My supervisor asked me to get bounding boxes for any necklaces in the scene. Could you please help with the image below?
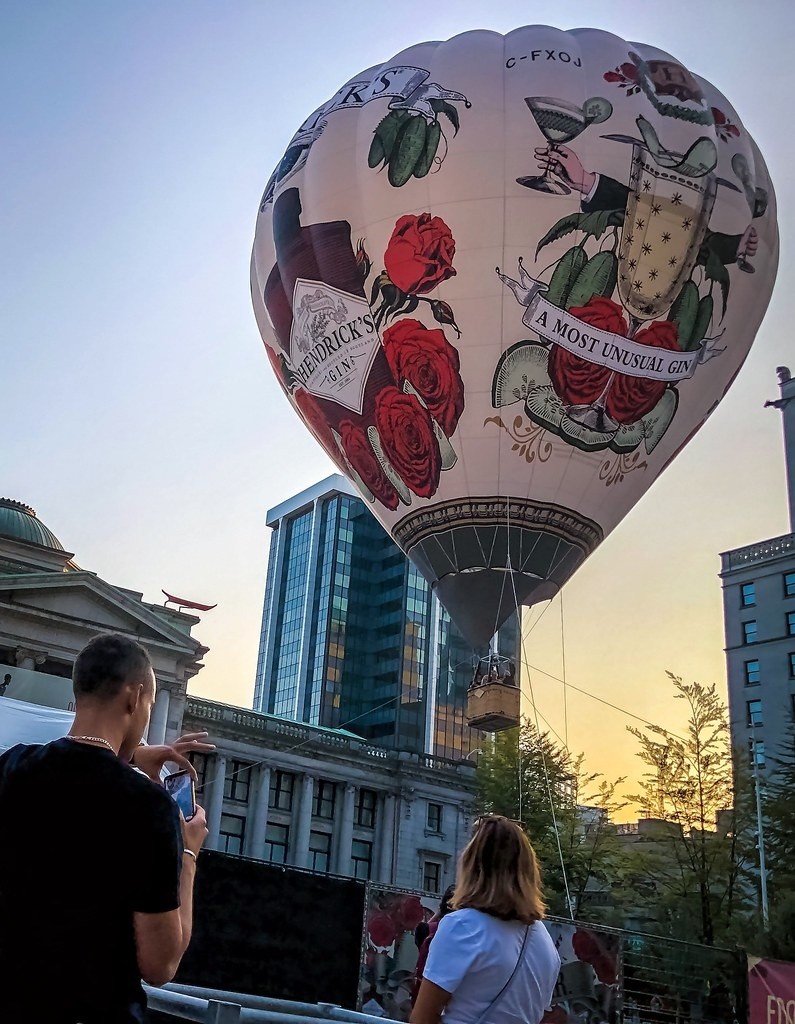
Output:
[66,735,114,751]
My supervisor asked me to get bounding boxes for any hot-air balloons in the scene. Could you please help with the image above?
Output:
[250,23,781,735]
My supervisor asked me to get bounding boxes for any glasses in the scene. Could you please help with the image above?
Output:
[477,815,526,837]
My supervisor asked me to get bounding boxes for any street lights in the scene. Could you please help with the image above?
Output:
[748,713,770,933]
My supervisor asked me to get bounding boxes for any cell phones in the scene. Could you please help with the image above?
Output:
[164,769,197,824]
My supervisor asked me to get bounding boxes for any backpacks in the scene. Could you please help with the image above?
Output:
[412,922,437,1011]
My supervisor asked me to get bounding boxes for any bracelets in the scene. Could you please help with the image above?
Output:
[183,848,197,862]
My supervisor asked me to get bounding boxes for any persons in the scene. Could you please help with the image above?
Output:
[469,659,516,687]
[0,634,215,1024]
[408,815,561,1024]
[412,884,457,1005]
